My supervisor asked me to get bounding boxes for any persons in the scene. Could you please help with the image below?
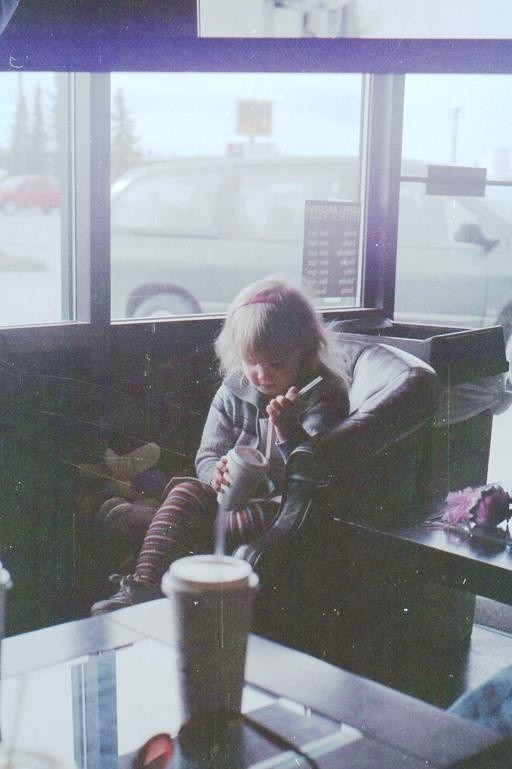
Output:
[87,274,348,616]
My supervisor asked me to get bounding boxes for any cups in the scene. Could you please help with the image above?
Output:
[159,554,258,750]
[213,447,268,512]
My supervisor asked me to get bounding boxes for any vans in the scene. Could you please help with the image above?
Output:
[105,157,512,383]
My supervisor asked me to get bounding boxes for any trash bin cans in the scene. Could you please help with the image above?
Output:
[327,316,509,492]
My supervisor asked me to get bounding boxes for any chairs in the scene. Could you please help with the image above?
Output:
[99,332,442,602]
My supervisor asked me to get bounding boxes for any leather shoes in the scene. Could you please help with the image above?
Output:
[90,574,159,614]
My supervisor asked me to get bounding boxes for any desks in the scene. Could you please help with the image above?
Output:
[0,598,504,769]
[334,487,512,680]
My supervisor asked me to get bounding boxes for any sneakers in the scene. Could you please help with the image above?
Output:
[103,503,160,533]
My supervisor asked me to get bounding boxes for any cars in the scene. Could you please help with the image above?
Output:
[1,173,57,212]
